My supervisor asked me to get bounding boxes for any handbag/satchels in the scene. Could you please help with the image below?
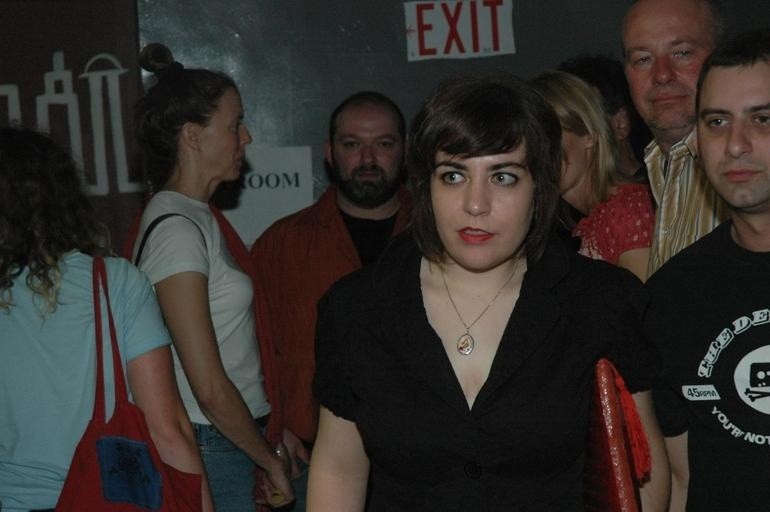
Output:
[56,401,203,512]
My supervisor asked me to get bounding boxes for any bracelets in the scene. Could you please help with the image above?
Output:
[271,448,288,454]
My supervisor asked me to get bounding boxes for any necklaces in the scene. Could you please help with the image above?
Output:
[435,254,521,357]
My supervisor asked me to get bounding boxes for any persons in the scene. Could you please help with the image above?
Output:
[305,76,670,510]
[559,53,652,199]
[524,66,654,287]
[250,90,415,510]
[131,41,296,511]
[644,29,770,510]
[621,0,729,283]
[0,125,216,512]
